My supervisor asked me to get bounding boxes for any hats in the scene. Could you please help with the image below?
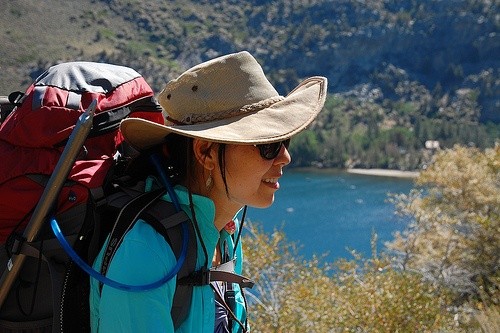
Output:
[118,48,327,156]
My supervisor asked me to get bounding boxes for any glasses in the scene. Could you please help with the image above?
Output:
[252,136,291,160]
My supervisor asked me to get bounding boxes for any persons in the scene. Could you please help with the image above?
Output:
[82,51,328,333]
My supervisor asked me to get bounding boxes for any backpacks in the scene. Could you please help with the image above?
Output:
[0,61,199,333]
